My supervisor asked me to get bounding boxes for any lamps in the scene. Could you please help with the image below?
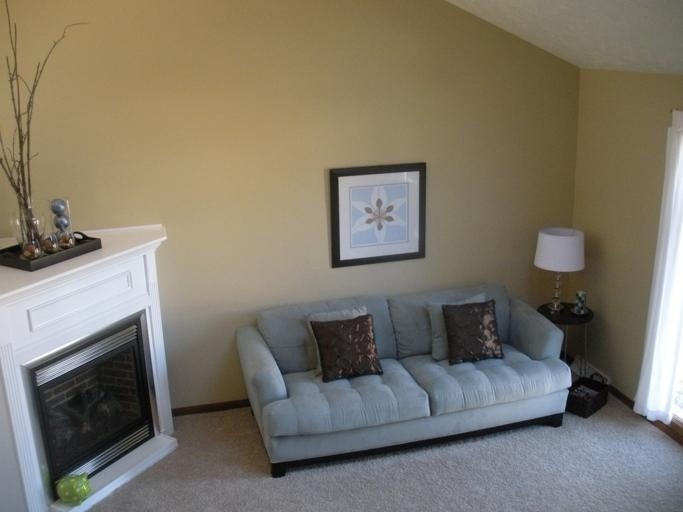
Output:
[532,226,586,317]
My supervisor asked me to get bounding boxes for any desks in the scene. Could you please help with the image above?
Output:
[538,299,592,368]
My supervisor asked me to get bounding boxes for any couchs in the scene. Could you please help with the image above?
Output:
[234,282,576,477]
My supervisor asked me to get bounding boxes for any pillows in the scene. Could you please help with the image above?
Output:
[441,298,503,367]
[310,311,384,381]
[422,293,486,361]
[304,304,366,379]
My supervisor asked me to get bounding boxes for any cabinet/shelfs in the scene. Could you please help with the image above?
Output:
[0,225,180,512]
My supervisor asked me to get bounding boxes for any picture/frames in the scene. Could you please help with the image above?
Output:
[328,162,428,269]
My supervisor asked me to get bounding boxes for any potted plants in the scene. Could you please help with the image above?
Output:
[10,192,46,256]
